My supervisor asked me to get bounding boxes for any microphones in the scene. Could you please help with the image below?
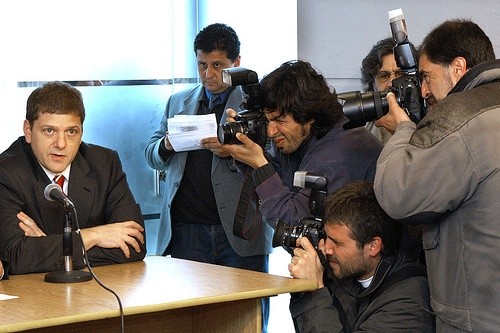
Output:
[44,183,75,209]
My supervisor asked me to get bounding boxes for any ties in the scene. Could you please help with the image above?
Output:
[209,95,220,109]
[53,175,65,193]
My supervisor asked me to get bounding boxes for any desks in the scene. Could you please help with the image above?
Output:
[0,255,319,333]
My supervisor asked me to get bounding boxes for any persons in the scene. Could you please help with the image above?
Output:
[290,19,500,333]
[222,60,385,259]
[0,22,275,333]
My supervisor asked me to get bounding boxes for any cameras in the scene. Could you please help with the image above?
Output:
[336,8,428,130]
[272,171,329,253]
[217,67,268,149]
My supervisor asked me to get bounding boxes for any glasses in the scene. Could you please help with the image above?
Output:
[376,69,402,82]
